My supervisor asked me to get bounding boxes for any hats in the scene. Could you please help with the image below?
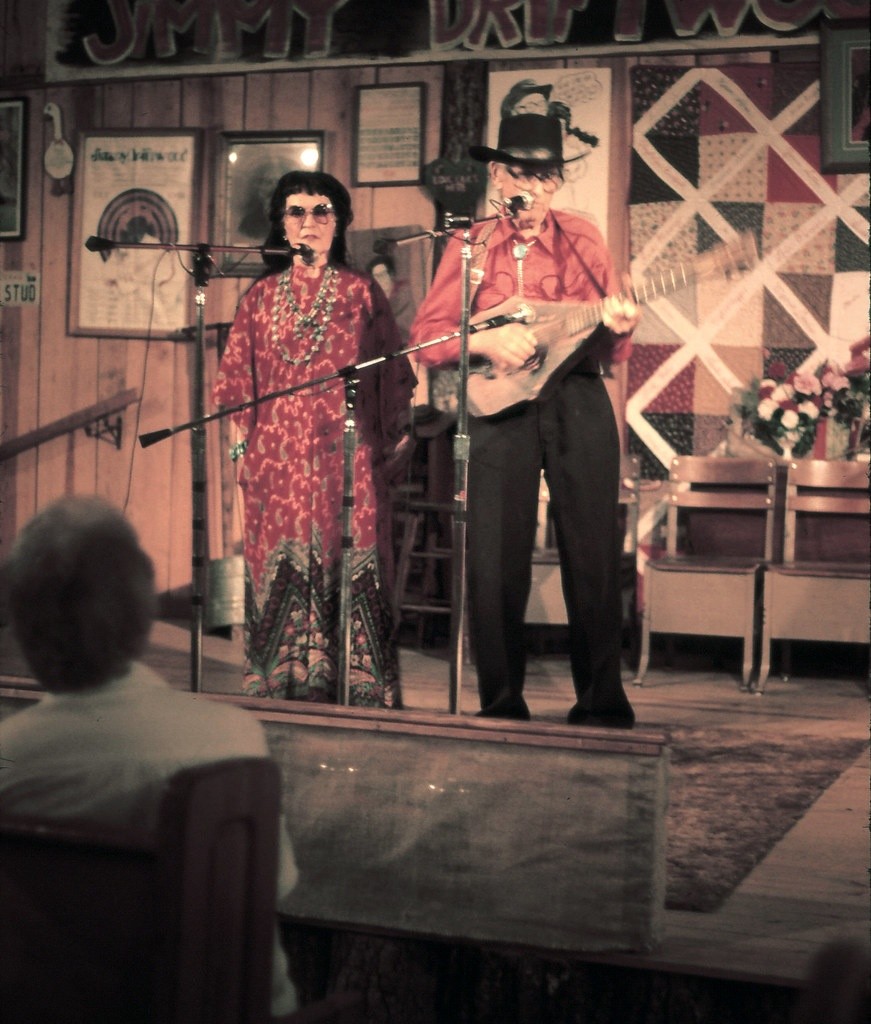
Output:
[470,113,591,168]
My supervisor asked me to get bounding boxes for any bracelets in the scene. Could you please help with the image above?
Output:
[229,440,248,463]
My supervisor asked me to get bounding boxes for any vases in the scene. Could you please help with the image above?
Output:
[773,429,800,458]
[848,422,862,457]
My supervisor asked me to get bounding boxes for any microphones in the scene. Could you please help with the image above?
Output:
[503,191,535,211]
[473,306,536,333]
[300,243,319,266]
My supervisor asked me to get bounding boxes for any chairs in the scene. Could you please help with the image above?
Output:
[632,455,777,689]
[756,458,871,693]
[510,461,643,626]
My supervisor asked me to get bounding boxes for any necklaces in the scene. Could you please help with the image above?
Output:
[511,236,537,296]
[271,265,338,364]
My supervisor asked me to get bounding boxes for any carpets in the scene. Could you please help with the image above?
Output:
[630,719,871,917]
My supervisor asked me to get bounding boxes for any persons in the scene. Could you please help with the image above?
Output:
[212,169,420,709]
[0,495,294,1024]
[411,116,638,730]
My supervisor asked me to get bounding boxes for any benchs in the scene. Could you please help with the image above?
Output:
[0,755,289,1024]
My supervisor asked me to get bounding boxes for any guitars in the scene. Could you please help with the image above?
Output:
[429,233,755,418]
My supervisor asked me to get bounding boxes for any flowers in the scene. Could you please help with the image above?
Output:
[795,339,870,415]
[750,377,821,439]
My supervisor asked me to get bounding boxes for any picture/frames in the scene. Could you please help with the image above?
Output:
[0,99,31,240]
[819,19,870,176]
[211,129,329,279]
[65,123,210,343]
[350,81,429,191]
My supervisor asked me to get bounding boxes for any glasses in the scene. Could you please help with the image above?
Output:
[505,165,564,194]
[284,203,335,223]
